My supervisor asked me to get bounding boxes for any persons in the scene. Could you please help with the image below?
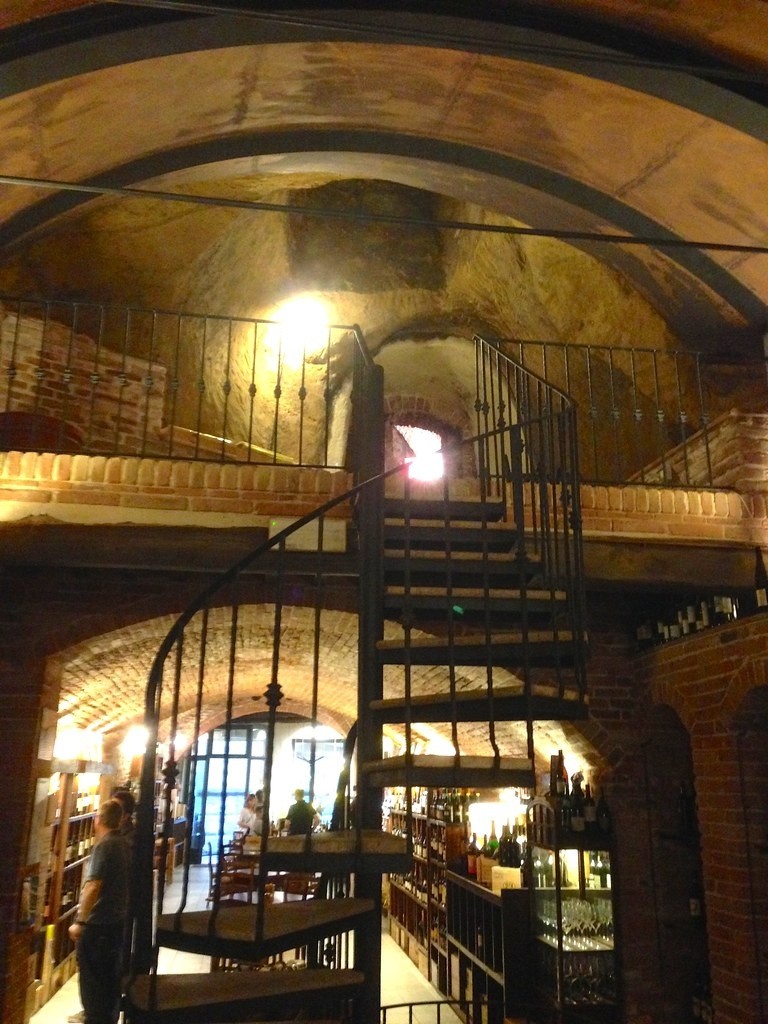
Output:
[237,789,264,839]
[67,792,141,1024]
[285,789,320,835]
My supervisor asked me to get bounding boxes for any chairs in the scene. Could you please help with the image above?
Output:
[208,830,320,905]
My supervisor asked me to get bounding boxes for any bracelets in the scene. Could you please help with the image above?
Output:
[73,920,86,925]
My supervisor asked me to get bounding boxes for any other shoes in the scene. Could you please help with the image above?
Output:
[68,1011,86,1023]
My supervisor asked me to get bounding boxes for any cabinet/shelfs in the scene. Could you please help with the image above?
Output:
[387,774,628,1024]
[33,758,116,1015]
